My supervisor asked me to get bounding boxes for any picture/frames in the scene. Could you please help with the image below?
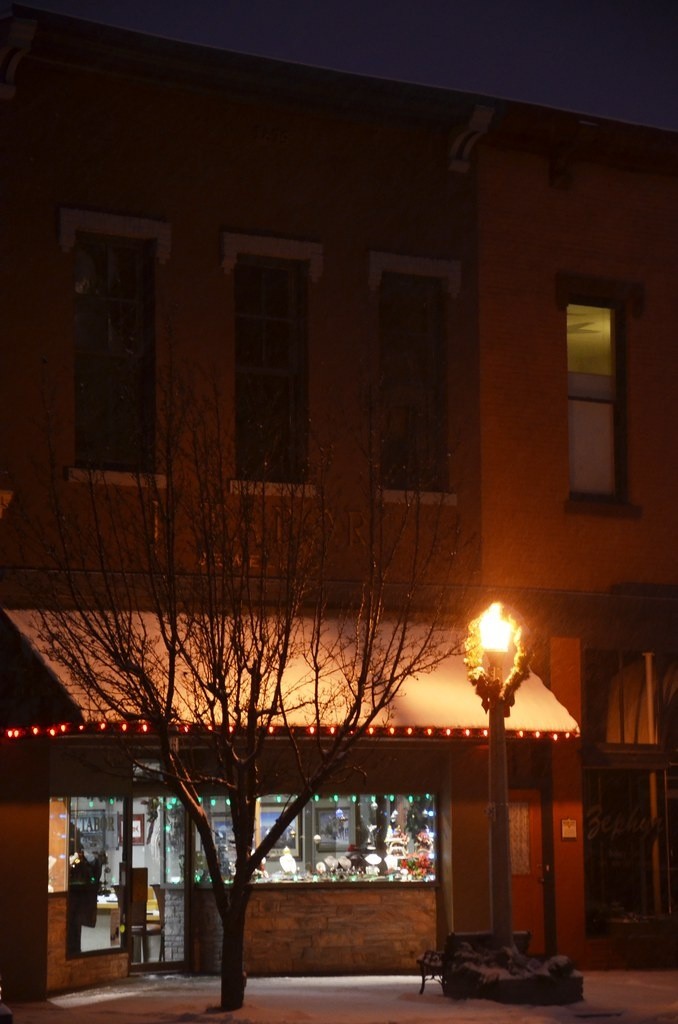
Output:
[255,802,302,861]
[117,812,145,846]
[207,812,237,862]
[314,807,361,852]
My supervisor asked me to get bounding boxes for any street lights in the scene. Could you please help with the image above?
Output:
[473,597,514,954]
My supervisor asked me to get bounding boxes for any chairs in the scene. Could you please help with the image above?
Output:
[111,885,162,962]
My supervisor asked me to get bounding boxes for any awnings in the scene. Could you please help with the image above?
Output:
[582,753,671,769]
[0,608,582,740]
[583,583,678,654]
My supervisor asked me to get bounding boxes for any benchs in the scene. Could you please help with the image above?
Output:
[417,930,531,997]
